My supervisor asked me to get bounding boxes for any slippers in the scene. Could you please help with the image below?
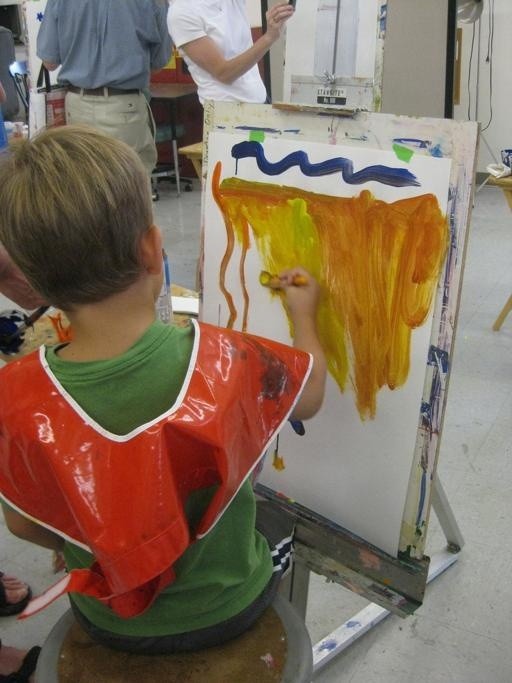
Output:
[0,640,41,683]
[0,571,32,617]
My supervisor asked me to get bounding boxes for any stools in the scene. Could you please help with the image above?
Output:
[32,591,315,683]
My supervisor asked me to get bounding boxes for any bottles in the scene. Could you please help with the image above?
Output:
[157,249,172,324]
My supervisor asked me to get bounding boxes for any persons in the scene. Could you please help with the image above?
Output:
[36,0,173,177]
[0,82,54,681]
[1,127,326,657]
[0,26,20,122]
[167,0,303,139]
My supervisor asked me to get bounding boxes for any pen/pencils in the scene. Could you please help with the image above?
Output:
[270,276,308,288]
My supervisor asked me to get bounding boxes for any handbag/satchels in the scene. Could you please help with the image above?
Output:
[28,63,67,139]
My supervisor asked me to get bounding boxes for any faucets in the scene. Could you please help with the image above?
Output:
[320,71,335,87]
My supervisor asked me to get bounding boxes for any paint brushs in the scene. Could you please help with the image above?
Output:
[3,306,49,344]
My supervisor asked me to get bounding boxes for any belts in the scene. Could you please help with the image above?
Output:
[67,84,139,96]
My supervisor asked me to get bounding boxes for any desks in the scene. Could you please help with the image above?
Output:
[149,81,197,196]
